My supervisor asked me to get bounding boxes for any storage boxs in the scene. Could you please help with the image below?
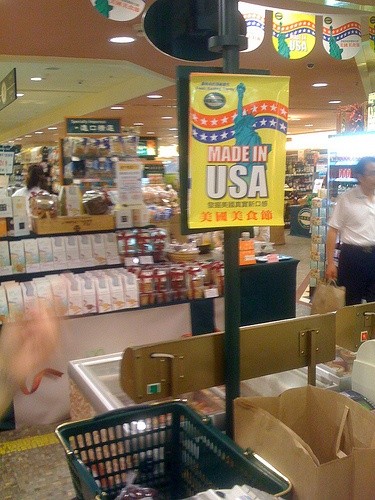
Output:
[31,214,116,234]
[233,384,375,500]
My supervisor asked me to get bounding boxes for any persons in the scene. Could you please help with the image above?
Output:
[0,294,64,417]
[11,165,50,230]
[326,157,375,306]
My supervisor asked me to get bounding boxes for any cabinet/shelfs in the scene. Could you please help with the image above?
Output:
[284,152,328,229]
[324,129,375,283]
[0,225,223,429]
